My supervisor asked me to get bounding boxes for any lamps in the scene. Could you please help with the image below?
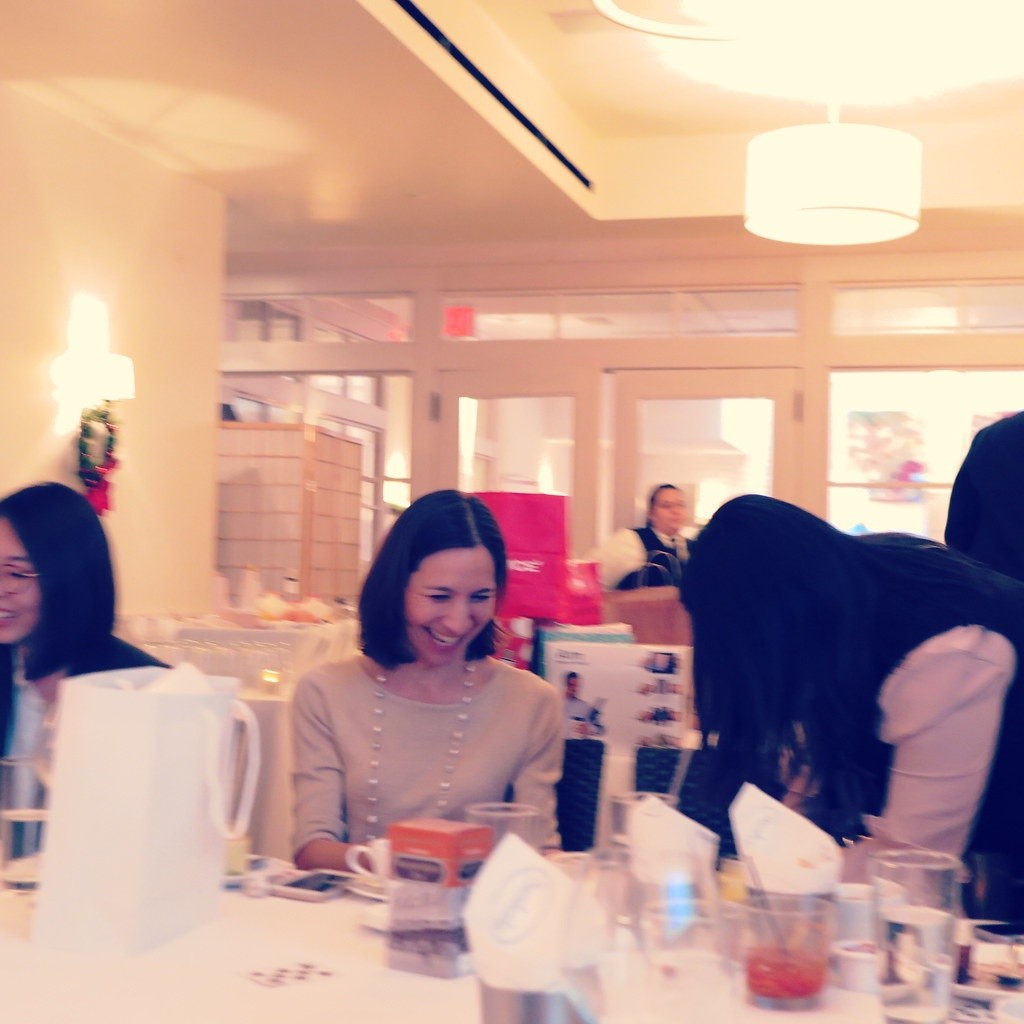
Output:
[743,107,924,245]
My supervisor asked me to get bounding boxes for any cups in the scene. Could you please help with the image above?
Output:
[0,755,46,891]
[464,801,543,855]
[603,792,679,916]
[994,995,1024,1024]
[836,882,876,937]
[876,905,954,984]
[346,837,391,878]
[744,896,837,1012]
[869,849,961,1024]
[647,896,744,1024]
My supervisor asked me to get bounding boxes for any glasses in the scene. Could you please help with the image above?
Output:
[0,564,45,595]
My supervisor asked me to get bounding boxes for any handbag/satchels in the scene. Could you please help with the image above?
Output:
[604,549,692,646]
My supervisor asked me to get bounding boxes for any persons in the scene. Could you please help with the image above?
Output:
[0,482,173,867]
[600,412,1024,936]
[287,491,565,875]
[561,671,606,741]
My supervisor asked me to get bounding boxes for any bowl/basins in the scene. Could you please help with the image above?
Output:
[830,939,956,989]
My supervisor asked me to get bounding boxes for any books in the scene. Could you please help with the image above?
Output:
[534,620,693,751]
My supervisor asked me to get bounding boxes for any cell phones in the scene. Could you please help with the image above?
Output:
[274,870,355,902]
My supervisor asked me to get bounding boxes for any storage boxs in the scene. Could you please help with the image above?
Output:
[387,819,496,981]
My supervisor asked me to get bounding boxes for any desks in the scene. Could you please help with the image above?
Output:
[0,865,1024,1024]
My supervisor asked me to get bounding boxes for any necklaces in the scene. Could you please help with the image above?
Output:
[365,656,476,845]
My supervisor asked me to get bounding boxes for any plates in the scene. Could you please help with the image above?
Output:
[339,882,391,901]
[219,855,292,887]
[255,620,295,630]
[947,918,1024,1000]
